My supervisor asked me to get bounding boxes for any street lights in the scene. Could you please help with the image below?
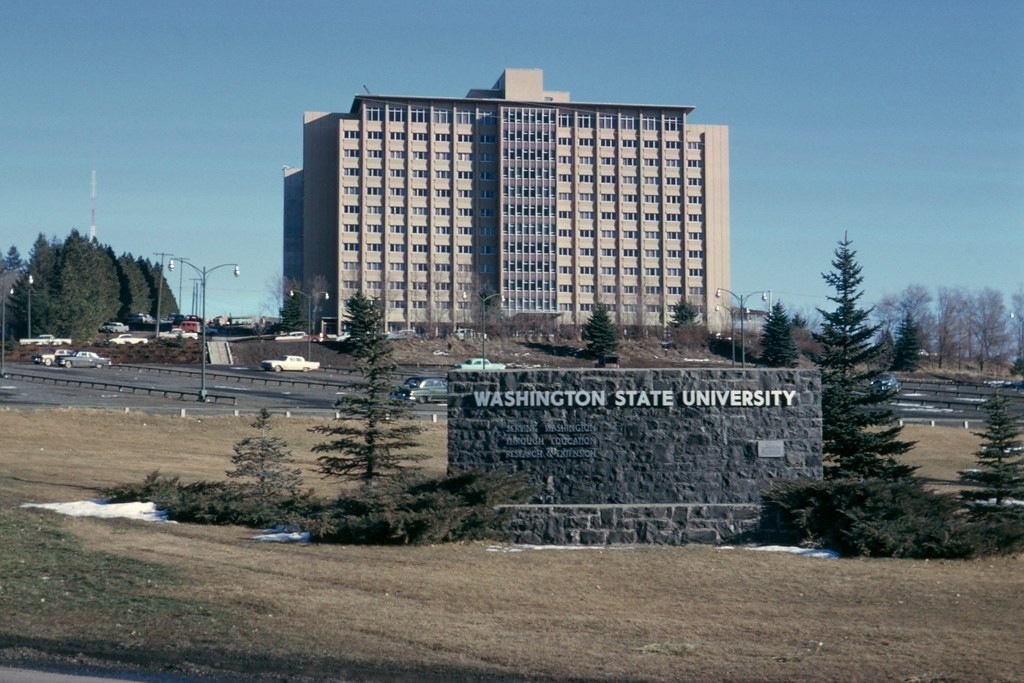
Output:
[289,290,330,361]
[167,257,240,402]
[460,290,505,370]
[713,287,767,367]
[9,273,33,338]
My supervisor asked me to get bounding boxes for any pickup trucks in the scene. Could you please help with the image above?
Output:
[108,333,149,344]
[159,327,198,341]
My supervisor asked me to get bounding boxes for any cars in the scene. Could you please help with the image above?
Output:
[261,355,321,371]
[869,373,903,397]
[200,324,219,335]
[335,332,352,342]
[31,349,112,368]
[99,322,130,333]
[452,328,487,341]
[389,376,447,404]
[134,312,198,324]
[39,334,55,340]
[275,330,309,341]
[453,358,506,372]
